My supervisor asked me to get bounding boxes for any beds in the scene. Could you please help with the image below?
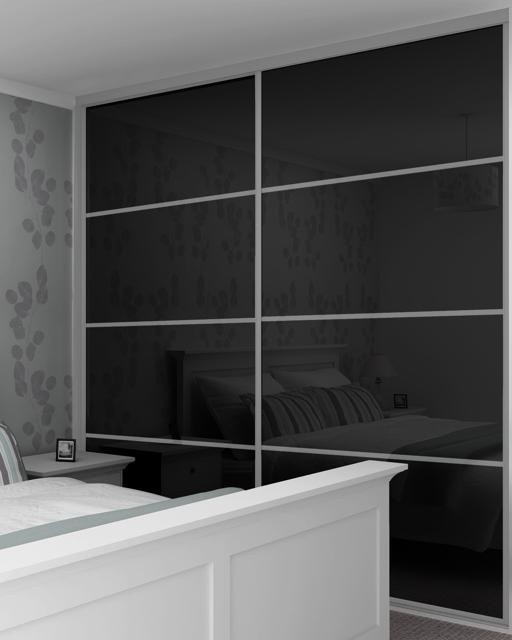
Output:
[0,433,409,639]
[167,343,500,492]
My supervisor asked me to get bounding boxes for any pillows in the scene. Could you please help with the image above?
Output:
[196,369,383,435]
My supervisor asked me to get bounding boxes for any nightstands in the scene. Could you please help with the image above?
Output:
[100,434,230,498]
[383,406,431,419]
[21,450,135,487]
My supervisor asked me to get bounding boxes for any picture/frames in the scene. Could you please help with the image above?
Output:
[394,393,408,410]
[55,437,76,461]
